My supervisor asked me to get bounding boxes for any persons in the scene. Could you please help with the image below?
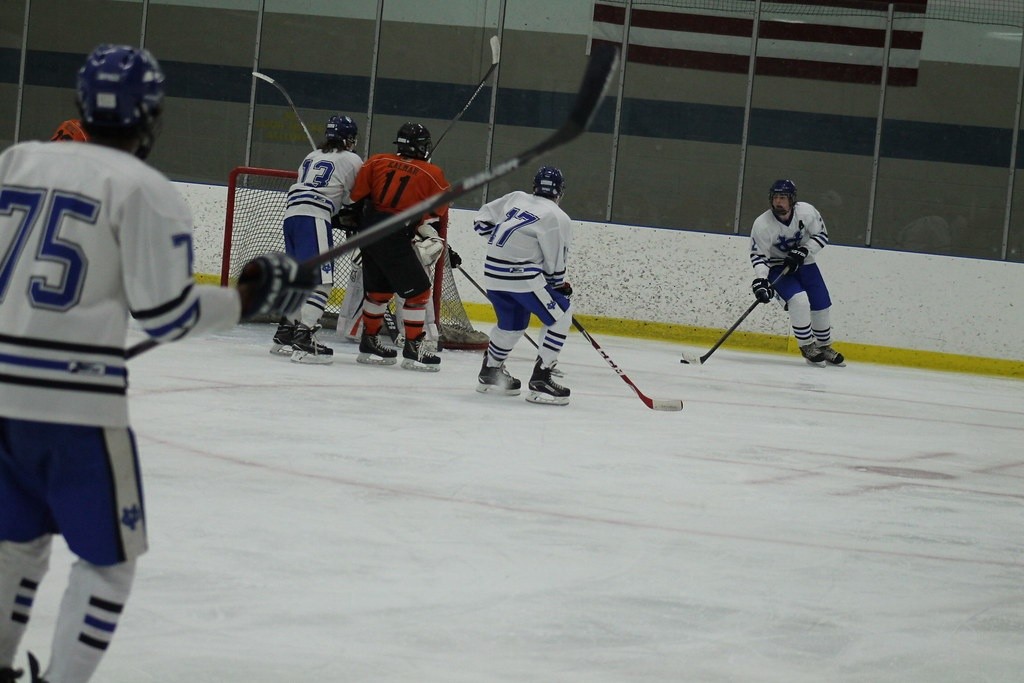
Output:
[270,115,363,364]
[351,122,450,372]
[0,43,309,683]
[51,119,88,143]
[473,166,574,405]
[751,180,847,368]
[337,215,461,352]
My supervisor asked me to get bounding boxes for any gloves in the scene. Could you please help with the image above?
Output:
[752,278,774,304]
[446,243,462,268]
[555,282,573,297]
[237,251,319,324]
[782,246,808,276]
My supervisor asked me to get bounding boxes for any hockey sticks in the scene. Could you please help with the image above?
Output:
[123,39,626,363]
[456,264,541,351]
[571,316,685,412]
[681,267,790,366]
[251,70,318,151]
[425,35,503,162]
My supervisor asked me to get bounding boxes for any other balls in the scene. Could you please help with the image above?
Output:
[680,360,689,364]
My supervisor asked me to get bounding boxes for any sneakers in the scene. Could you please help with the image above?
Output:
[401,331,441,372]
[799,342,827,367]
[820,345,847,367]
[291,322,334,364]
[355,325,397,365]
[476,351,522,395]
[270,316,299,355]
[526,359,571,405]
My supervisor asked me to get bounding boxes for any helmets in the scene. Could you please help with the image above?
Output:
[324,115,359,152]
[533,166,565,199]
[768,179,798,214]
[77,42,180,150]
[393,121,433,160]
